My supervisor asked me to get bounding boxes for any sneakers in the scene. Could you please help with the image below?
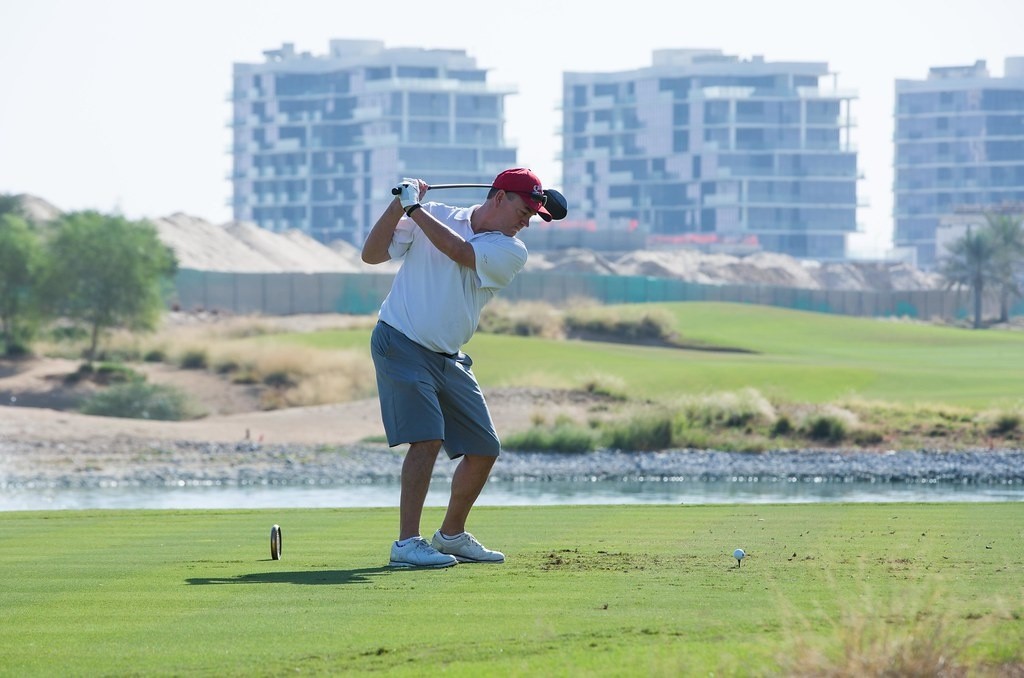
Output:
[388,537,458,569]
[431,528,505,563]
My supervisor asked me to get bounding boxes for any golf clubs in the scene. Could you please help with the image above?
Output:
[392,183,567,221]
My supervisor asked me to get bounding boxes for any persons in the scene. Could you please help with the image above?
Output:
[360,167,548,569]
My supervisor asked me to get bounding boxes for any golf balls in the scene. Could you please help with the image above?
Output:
[734,549,745,560]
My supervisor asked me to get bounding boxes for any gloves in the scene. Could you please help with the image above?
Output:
[395,177,428,212]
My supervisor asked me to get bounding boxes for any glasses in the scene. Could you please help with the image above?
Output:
[505,189,548,206]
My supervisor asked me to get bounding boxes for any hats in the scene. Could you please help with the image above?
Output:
[492,168,553,223]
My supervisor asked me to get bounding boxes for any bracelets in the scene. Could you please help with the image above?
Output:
[406,203,422,218]
